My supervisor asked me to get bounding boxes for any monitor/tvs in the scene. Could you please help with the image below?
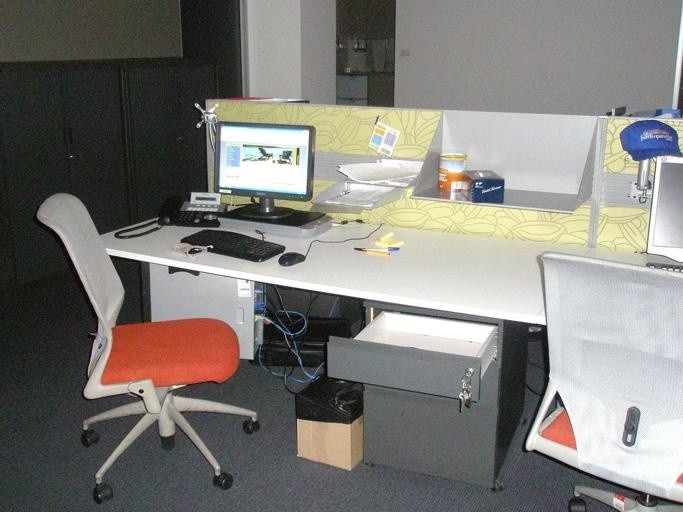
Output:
[648,156,683,262]
[215,120,326,227]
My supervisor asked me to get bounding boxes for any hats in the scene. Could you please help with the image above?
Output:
[619,119,683,160]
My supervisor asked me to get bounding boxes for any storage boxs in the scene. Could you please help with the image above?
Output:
[296,378,363,471]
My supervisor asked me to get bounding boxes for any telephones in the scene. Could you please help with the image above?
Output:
[115,192,228,239]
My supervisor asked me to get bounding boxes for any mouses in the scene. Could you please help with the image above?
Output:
[278,252,305,265]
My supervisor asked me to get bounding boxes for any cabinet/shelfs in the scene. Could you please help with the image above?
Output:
[0,59,131,286]
[337,73,393,107]
[124,59,217,225]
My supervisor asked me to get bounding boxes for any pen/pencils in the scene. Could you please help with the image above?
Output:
[362,247,400,251]
[354,248,390,256]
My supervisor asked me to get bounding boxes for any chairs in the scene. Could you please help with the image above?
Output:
[524,251,683,512]
[36,192,260,503]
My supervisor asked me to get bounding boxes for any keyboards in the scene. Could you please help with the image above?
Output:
[645,263,681,272]
[180,229,285,262]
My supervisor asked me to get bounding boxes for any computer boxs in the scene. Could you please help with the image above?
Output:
[140,260,267,361]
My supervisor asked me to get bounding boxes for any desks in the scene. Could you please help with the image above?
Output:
[99,97,683,488]
